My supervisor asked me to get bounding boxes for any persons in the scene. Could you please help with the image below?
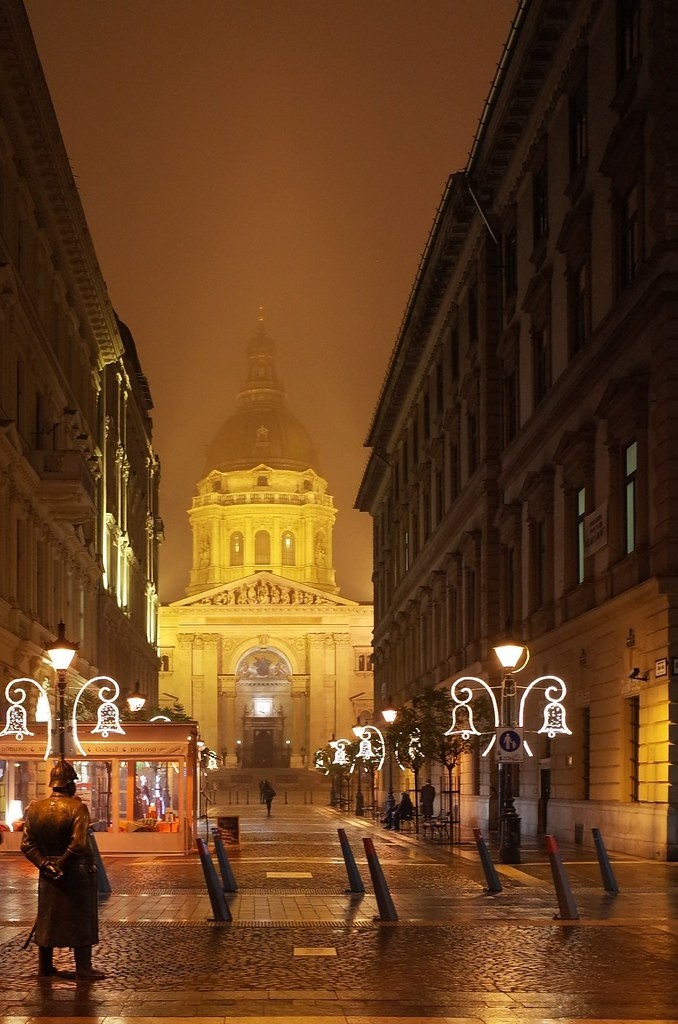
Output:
[258,780,265,802]
[392,792,413,830]
[380,803,401,829]
[264,780,268,784]
[264,782,274,815]
[420,779,436,821]
[19,759,106,981]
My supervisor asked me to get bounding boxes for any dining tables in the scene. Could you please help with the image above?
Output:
[0,810,180,833]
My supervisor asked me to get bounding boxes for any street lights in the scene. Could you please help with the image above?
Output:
[0,618,126,760]
[444,643,574,861]
[126,679,170,723]
[197,734,219,818]
[315,734,349,807]
[353,708,427,834]
[329,716,381,815]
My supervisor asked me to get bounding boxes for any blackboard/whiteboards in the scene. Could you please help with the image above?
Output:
[215,815,241,846]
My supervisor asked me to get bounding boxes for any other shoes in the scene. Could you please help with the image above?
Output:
[378,814,437,833]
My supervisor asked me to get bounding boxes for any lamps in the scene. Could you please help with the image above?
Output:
[285,534,291,547]
[234,536,240,553]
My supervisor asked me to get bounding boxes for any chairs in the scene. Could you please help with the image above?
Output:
[331,797,450,839]
